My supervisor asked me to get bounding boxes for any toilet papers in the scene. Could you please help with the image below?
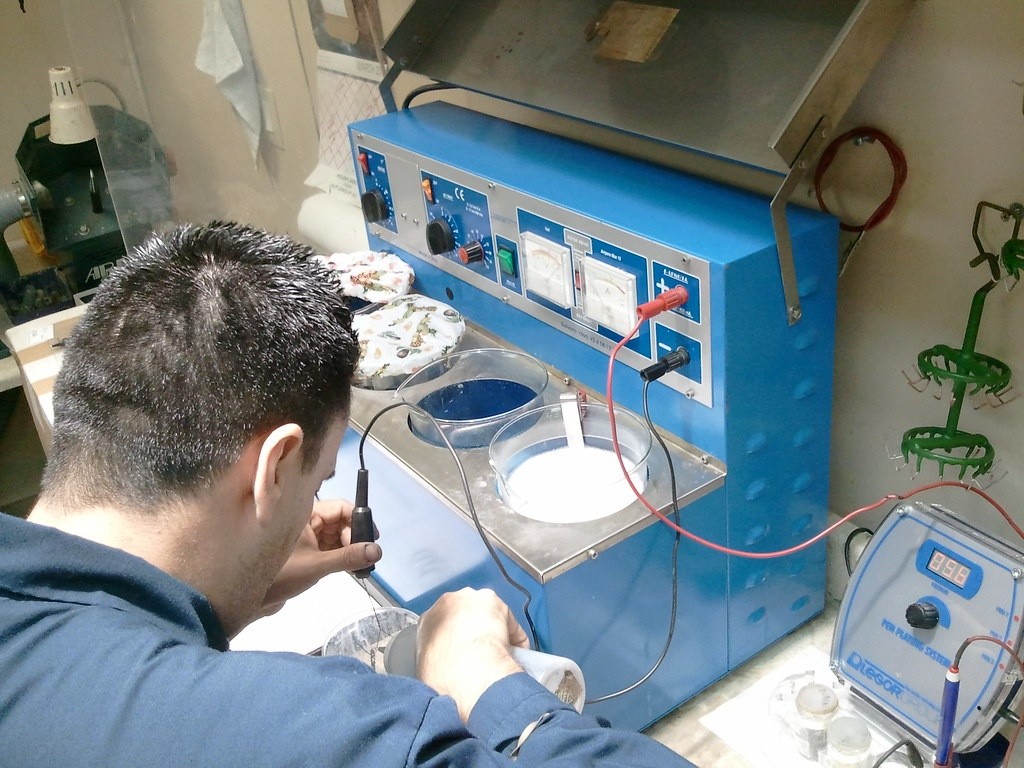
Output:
[295,191,370,255]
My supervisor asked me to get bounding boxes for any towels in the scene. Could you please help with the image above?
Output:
[192,0,265,175]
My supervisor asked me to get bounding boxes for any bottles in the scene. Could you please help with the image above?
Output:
[379,625,586,718]
[792,684,871,768]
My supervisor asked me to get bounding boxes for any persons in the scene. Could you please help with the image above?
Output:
[0,220,699,767]
